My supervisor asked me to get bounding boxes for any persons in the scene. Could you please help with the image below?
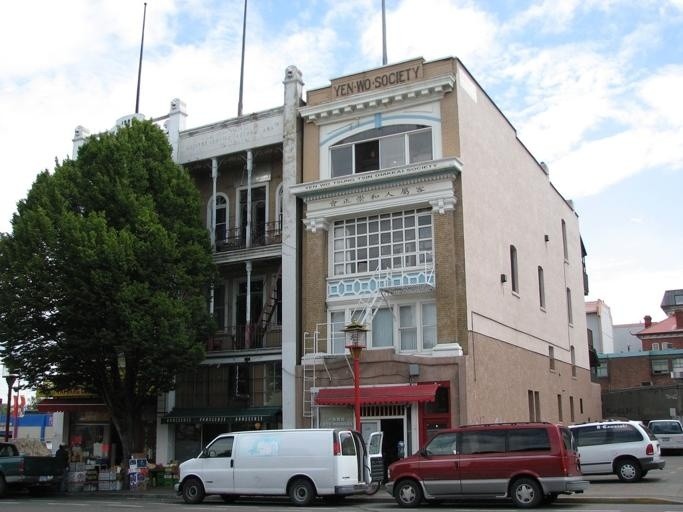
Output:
[56,442,71,492]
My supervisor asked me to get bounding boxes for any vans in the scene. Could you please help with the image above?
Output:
[385,422,590,508]
[567,419,666,481]
[174,429,384,507]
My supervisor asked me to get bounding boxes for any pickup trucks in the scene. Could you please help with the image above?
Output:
[0,443,65,496]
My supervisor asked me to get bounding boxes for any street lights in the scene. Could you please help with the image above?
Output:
[342,320,368,437]
[2,375,18,441]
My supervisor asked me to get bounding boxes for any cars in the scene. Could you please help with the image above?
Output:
[647,418,683,449]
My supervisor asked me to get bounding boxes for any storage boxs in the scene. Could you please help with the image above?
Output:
[64,458,149,491]
